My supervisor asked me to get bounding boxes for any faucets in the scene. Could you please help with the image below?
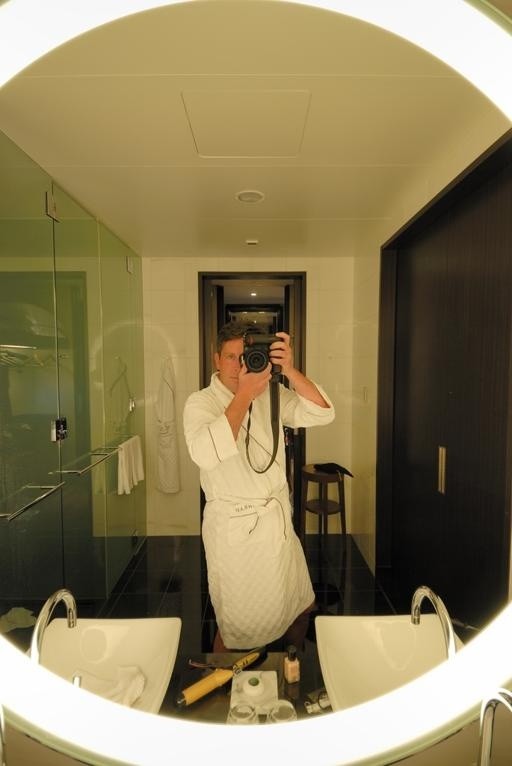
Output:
[413,586,456,659]
[475,689,512,765]
[28,587,79,662]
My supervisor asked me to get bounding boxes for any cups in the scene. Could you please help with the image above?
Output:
[226,702,259,724]
[266,699,298,724]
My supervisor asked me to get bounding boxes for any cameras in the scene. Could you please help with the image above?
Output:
[239,332,284,373]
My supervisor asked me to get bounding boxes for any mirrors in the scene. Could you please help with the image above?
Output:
[0,0,512,766]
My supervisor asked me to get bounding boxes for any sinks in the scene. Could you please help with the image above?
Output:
[32,618,180,714]
[313,612,464,712]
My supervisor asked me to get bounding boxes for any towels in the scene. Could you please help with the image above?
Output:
[66,665,145,708]
[117,435,145,495]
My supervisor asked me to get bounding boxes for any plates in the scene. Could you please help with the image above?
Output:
[230,670,279,715]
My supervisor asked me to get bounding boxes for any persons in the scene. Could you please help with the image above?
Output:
[184,319,336,654]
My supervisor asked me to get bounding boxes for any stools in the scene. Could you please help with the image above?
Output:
[300,464,347,549]
